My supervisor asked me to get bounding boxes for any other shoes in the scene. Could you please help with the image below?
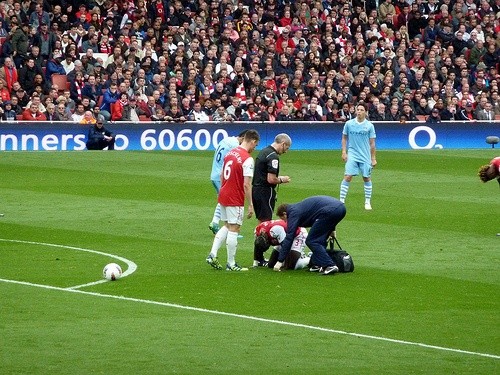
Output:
[364,203,372,210]
[258,259,269,266]
[308,252,313,257]
[301,252,306,258]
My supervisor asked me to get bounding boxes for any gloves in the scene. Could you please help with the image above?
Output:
[273,261,282,272]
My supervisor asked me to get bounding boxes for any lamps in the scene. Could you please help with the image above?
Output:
[486,135,499,149]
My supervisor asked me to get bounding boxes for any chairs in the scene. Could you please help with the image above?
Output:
[51,73,66,91]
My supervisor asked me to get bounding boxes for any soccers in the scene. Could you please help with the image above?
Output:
[103,263,122,280]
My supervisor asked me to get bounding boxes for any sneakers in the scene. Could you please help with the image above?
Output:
[322,266,339,275]
[226,263,248,272]
[209,222,220,234]
[309,265,322,272]
[206,253,223,270]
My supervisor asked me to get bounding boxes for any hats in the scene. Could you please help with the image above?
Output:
[270,225,286,243]
[466,102,472,107]
[185,90,193,94]
[97,118,103,124]
[254,235,270,252]
[433,108,439,113]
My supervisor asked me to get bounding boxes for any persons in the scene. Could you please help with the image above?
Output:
[273,195,346,276]
[0,0,500,122]
[252,134,292,267]
[208,129,249,239]
[86,119,115,151]
[478,156,500,236]
[254,219,313,270]
[339,103,378,210]
[207,129,261,272]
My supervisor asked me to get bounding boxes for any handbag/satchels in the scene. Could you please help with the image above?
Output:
[325,236,354,273]
